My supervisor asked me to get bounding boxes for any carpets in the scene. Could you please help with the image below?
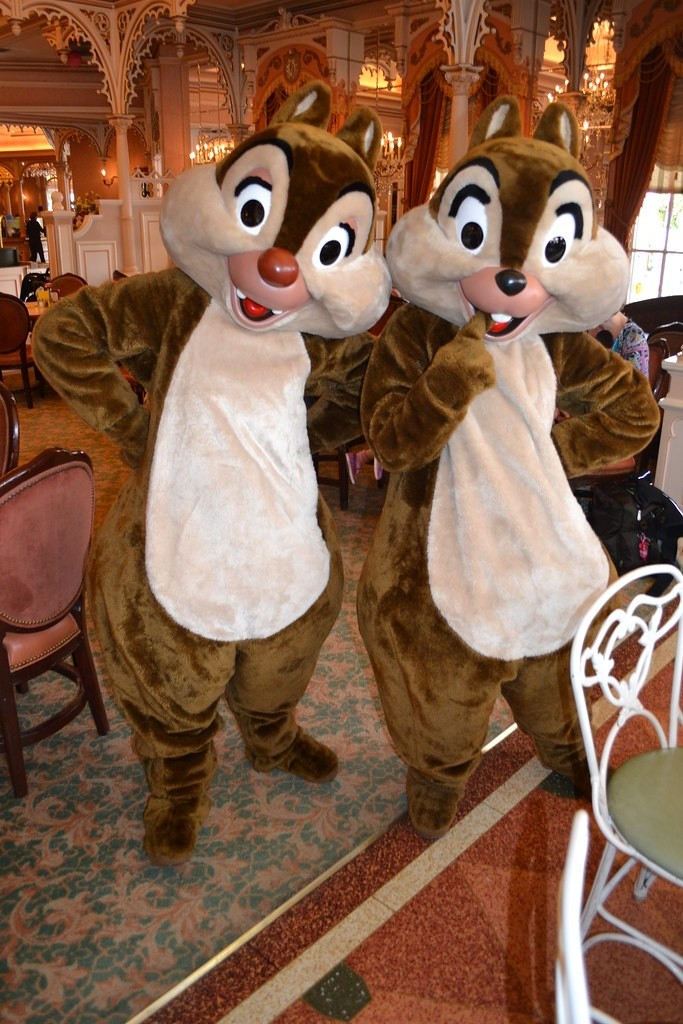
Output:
[124,592,682,1024]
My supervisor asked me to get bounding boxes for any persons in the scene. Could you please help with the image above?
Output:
[345,448,384,484]
[550,305,648,567]
[26,212,45,262]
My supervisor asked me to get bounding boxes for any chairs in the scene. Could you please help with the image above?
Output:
[551,564,683,1024]
[0,267,134,480]
[1,443,112,798]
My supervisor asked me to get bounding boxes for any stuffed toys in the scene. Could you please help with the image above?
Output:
[358,96,661,837]
[30,79,392,863]
